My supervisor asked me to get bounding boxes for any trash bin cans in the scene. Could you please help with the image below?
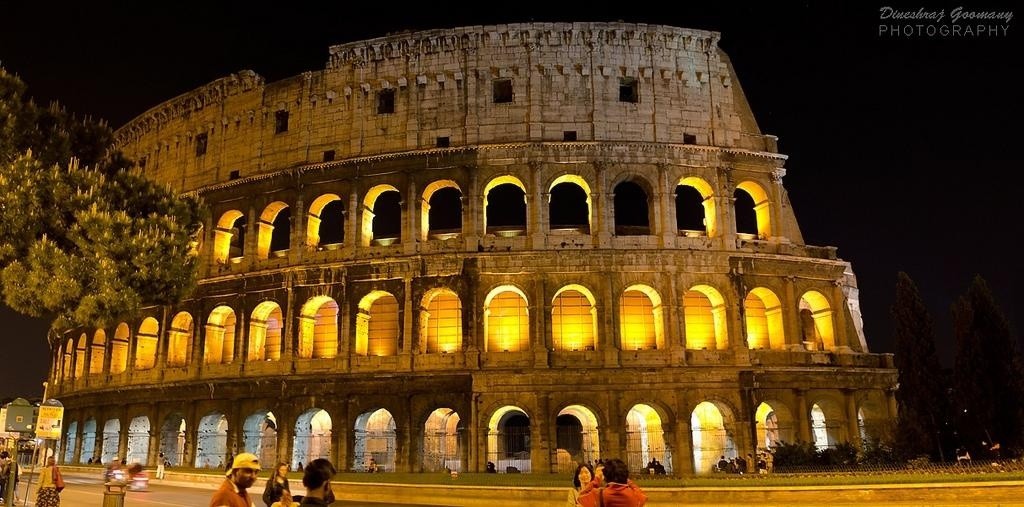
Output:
[103,482,126,507]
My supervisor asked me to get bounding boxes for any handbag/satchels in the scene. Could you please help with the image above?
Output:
[55,472,65,491]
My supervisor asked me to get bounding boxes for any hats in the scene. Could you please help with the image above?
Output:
[226,453,261,476]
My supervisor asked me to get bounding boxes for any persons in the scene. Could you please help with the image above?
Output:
[0,450,141,506]
[715,452,767,474]
[163,457,171,467]
[487,461,494,472]
[644,456,666,475]
[155,452,165,479]
[587,458,608,470]
[576,458,648,506]
[209,452,336,506]
[368,458,376,472]
[565,462,595,506]
